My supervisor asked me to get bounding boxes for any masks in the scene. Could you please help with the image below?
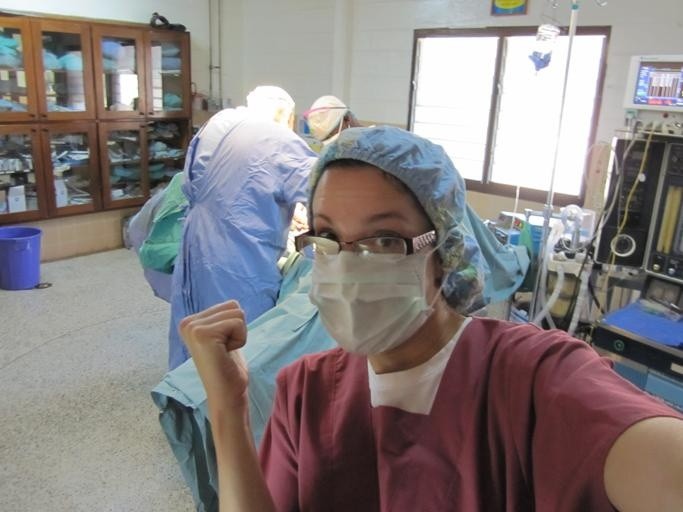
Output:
[307,248,435,357]
[321,133,340,152]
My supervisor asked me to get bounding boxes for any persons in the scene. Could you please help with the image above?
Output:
[298,95,378,144]
[175,119,682,512]
[168,84,327,367]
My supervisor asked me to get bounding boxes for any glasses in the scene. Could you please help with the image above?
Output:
[293,229,437,268]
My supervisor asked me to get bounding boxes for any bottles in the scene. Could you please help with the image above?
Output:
[526,23,560,71]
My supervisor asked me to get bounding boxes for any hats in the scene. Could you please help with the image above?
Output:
[307,125,491,317]
[247,86,295,131]
[306,95,350,143]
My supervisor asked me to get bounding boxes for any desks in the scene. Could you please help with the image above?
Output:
[591,298,683,415]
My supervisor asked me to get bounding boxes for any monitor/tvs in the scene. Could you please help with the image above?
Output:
[624,55,683,112]
